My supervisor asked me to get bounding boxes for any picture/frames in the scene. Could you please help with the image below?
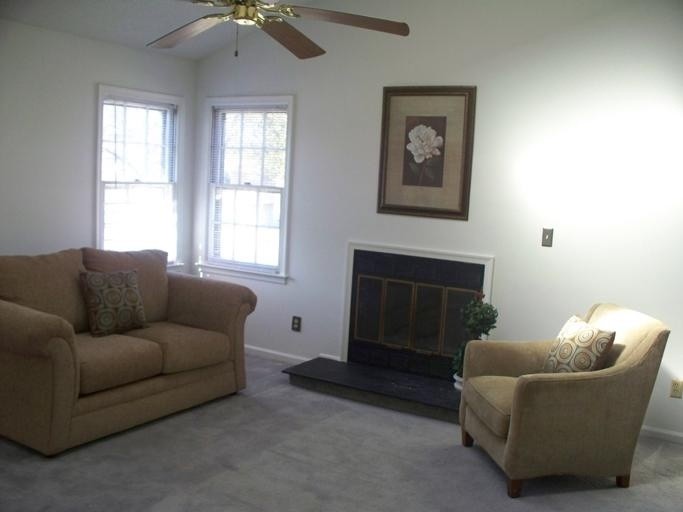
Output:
[376,84,477,221]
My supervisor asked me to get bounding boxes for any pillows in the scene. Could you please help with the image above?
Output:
[542,312,618,373]
[77,267,151,339]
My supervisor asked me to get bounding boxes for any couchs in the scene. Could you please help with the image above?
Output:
[1,249,258,458]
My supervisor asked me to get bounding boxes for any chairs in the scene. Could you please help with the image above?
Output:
[458,302,671,499]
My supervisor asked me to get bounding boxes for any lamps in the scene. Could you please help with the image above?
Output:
[231,3,268,29]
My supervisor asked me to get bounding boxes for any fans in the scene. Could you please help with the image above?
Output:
[144,2,411,59]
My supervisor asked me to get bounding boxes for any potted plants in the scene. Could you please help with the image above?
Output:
[450,300,498,392]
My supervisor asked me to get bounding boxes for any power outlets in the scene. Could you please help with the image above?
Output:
[670,378,683,398]
